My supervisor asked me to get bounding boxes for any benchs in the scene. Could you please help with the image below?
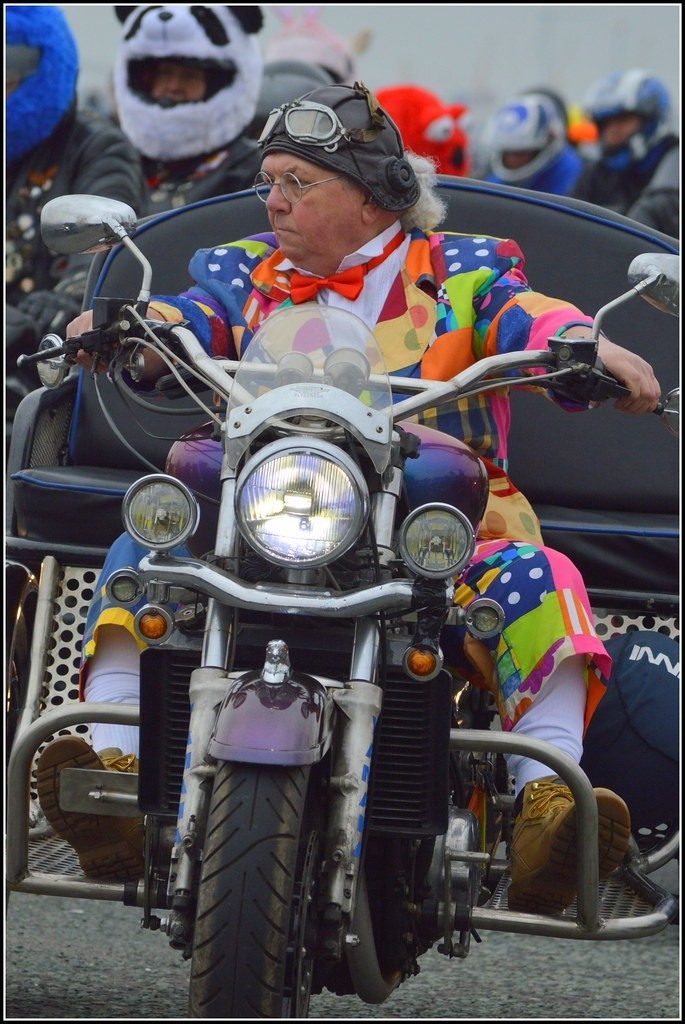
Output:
[11,171,680,598]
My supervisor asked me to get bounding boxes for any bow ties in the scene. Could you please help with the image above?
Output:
[288,229,405,305]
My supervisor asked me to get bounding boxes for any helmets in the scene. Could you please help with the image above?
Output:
[7,5,79,167]
[488,85,569,186]
[583,67,670,178]
[110,6,263,162]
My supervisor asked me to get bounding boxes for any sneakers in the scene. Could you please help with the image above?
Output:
[37,736,148,884]
[507,771,631,915]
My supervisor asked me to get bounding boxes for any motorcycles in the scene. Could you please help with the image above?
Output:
[6,174,681,1020]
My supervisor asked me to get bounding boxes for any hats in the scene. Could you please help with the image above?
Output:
[257,81,421,211]
[376,85,470,181]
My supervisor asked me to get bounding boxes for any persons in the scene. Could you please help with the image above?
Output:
[8,5,678,351]
[36,82,661,916]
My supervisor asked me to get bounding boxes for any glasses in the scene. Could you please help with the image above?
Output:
[254,100,346,147]
[251,171,345,204]
